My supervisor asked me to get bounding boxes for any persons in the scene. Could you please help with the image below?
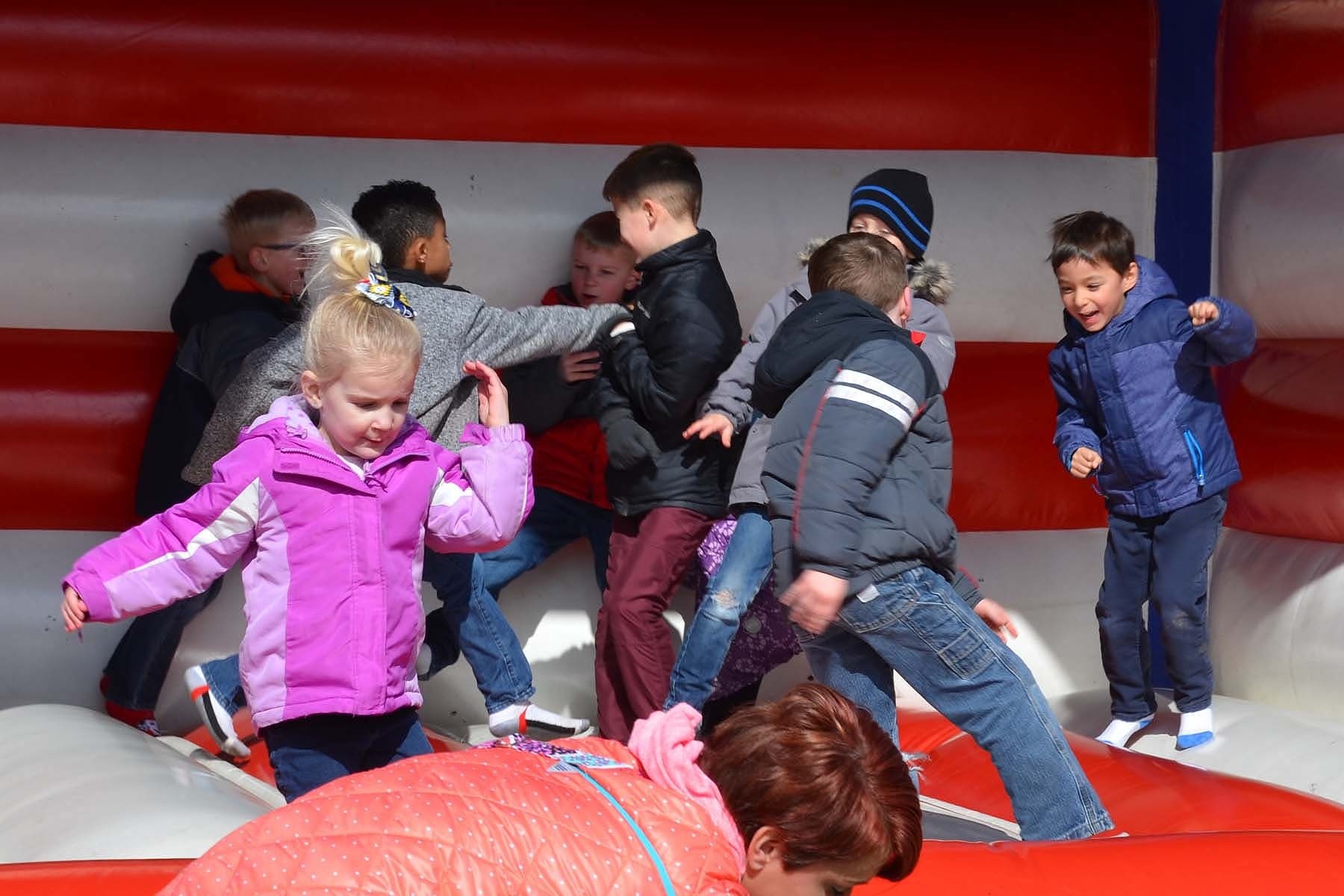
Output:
[753,231,1110,839]
[412,212,641,679]
[99,187,315,743]
[62,238,534,802]
[1048,210,1256,751]
[157,683,923,896]
[181,178,629,760]
[582,142,738,746]
[664,169,957,754]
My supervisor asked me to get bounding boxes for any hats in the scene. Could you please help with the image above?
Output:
[845,165,934,262]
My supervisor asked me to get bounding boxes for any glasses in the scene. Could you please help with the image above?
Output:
[253,244,313,261]
